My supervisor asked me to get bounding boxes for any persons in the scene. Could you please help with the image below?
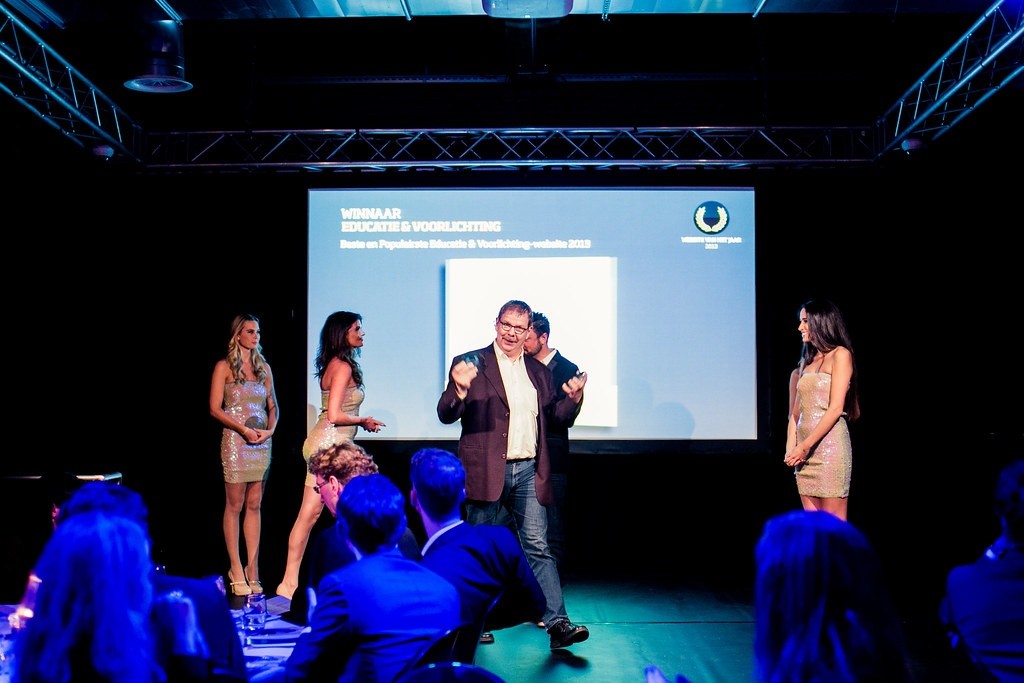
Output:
[276,312,386,600]
[942,464,1024,683]
[645,510,958,683]
[437,300,589,648]
[9,482,249,683]
[411,449,547,634]
[286,473,460,683]
[307,440,417,572]
[784,299,860,522]
[211,317,279,595]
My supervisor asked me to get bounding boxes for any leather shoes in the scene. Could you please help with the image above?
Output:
[479,631,493,641]
[549,621,589,648]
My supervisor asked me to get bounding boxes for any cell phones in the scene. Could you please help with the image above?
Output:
[576,372,585,378]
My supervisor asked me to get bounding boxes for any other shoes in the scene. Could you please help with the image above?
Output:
[537,620,545,626]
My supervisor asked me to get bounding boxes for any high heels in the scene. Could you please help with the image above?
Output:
[243,565,262,593]
[228,567,251,596]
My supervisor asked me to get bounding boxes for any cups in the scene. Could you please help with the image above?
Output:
[244,595,266,630]
[231,611,244,648]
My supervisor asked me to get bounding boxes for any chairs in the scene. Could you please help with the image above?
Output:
[396,593,507,683]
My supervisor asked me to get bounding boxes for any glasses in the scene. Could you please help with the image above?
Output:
[314,480,329,494]
[498,318,529,335]
